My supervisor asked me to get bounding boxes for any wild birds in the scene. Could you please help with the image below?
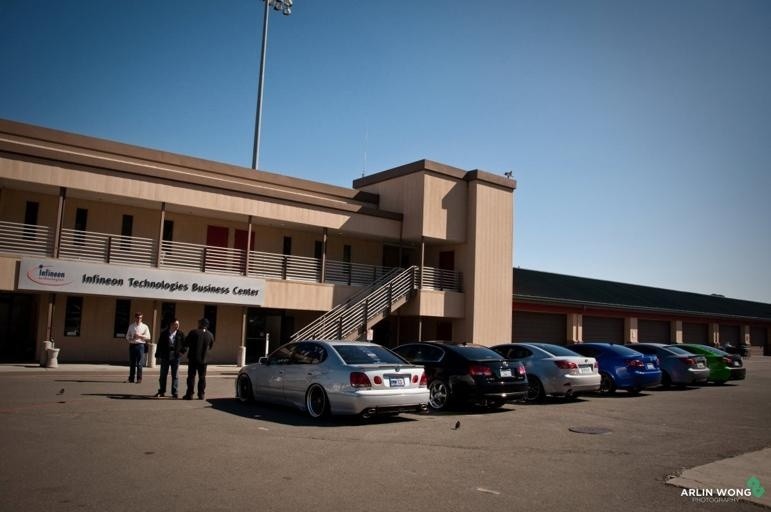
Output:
[455,421,460,431]
[60,388,64,394]
[505,171,513,179]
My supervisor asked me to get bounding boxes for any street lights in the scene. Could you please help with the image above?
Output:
[250,1,292,171]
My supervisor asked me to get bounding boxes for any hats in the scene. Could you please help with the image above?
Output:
[199,319,209,325]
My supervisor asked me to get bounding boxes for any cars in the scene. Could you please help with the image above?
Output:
[390,340,529,419]
[627,341,709,392]
[672,342,747,389]
[233,338,430,421]
[487,341,601,406]
[568,340,663,393]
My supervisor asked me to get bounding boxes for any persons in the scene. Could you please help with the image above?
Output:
[154,317,187,400]
[183,316,217,400]
[125,310,153,385]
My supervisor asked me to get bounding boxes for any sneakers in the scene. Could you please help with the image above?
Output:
[124,380,141,383]
[154,393,203,400]
